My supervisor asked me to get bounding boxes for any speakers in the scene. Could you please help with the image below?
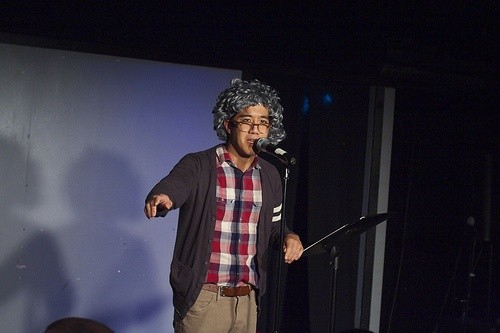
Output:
[463,174,500,247]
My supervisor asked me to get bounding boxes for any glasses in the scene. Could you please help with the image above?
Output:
[231,122,269,134]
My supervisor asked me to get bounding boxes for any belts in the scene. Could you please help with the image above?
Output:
[202,284,250,297]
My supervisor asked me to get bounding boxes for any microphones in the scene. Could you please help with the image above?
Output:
[257,138,296,164]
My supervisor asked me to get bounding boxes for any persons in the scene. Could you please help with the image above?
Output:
[144,78,304,333]
